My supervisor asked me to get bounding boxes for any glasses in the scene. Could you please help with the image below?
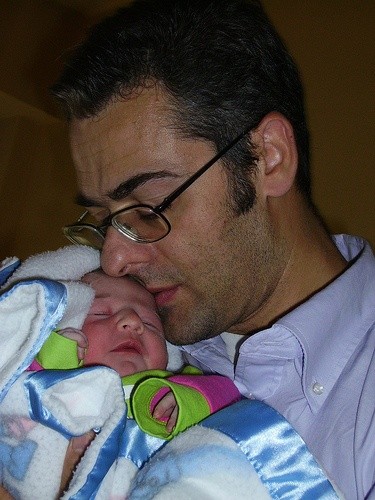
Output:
[61,113,269,248]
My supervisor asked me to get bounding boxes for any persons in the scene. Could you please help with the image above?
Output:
[0,244,241,500]
[50,1,375,500]
